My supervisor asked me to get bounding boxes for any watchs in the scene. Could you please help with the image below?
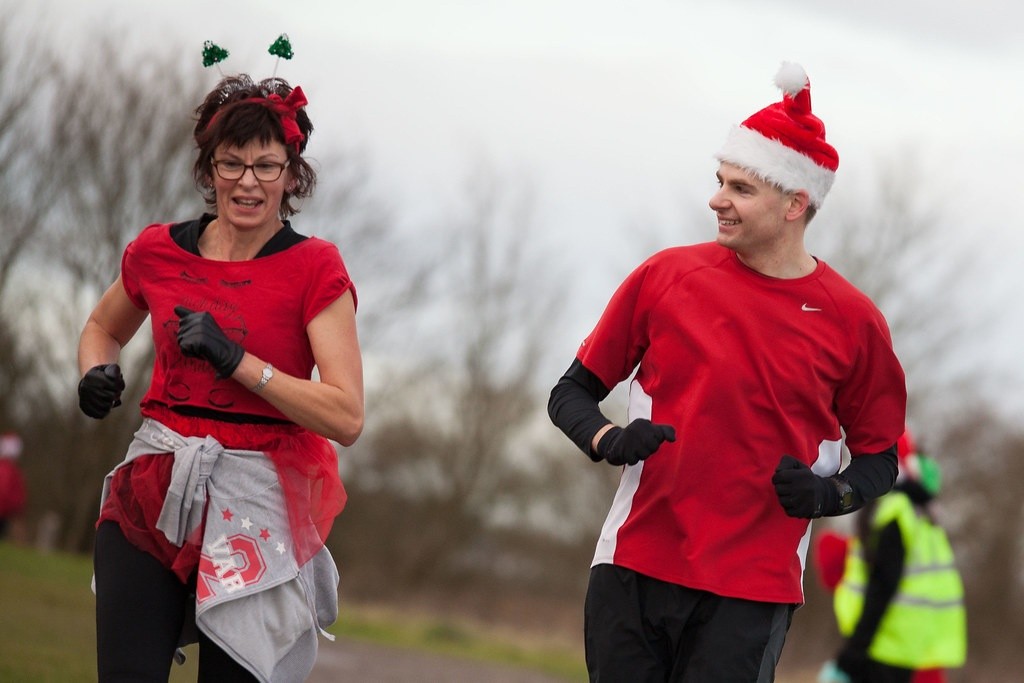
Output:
[250,364,273,393]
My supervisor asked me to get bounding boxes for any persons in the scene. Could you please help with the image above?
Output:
[0,431,27,540]
[815,426,970,683]
[547,67,908,683]
[77,73,361,683]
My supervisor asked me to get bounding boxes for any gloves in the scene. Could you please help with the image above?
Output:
[173,305,245,379]
[773,456,839,519]
[78,364,126,421]
[597,418,674,466]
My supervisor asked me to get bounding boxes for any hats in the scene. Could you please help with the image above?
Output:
[716,61,838,210]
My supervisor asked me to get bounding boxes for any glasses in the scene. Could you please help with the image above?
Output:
[206,153,290,183]
